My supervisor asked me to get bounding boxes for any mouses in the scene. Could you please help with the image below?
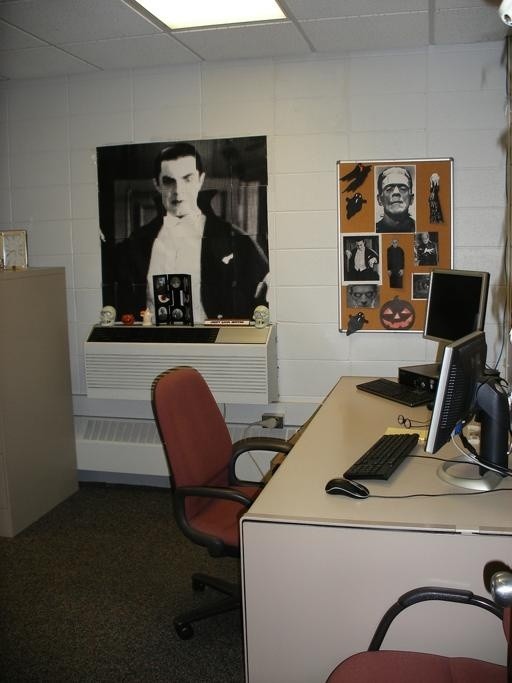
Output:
[325,477,369,499]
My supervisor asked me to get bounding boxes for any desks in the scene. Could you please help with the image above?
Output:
[239,374,512,682]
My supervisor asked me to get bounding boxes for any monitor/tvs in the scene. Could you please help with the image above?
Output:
[423,331,510,492]
[423,270,490,363]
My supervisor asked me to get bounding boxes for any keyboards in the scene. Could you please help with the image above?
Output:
[343,433,420,480]
[356,378,434,407]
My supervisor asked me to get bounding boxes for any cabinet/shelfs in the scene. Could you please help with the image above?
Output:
[0,265,80,538]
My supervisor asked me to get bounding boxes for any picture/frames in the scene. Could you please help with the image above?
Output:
[337,157,455,335]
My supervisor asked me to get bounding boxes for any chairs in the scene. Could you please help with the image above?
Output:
[327,559,512,683]
[152,365,296,636]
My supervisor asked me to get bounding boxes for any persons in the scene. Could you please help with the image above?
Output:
[416,234,436,264]
[386,238,404,286]
[375,165,415,235]
[348,285,379,309]
[100,143,269,322]
[345,240,377,280]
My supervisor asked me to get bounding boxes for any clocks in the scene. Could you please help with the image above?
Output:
[1,229,29,271]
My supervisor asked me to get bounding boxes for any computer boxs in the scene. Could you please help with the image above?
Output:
[398,363,500,390]
